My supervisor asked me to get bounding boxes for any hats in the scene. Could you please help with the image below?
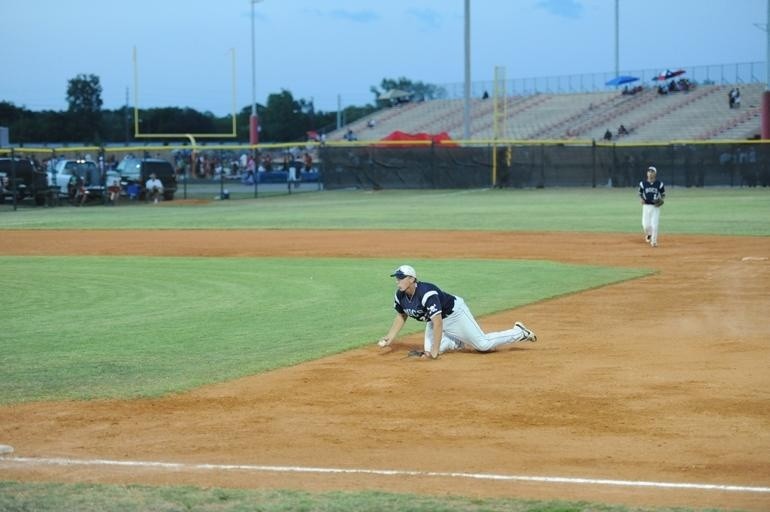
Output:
[390,265,416,279]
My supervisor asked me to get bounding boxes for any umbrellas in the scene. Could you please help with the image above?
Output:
[341,128,358,141]
[374,87,414,100]
[306,130,322,142]
[367,117,376,127]
[145,172,164,205]
[652,69,686,82]
[604,74,641,87]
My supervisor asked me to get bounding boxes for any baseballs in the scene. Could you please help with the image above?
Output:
[379,339,386,346]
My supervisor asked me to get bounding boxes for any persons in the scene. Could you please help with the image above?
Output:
[319,131,327,146]
[729,87,736,107]
[382,98,410,109]
[124,151,161,159]
[482,89,488,100]
[68,167,90,207]
[603,128,614,141]
[171,146,313,187]
[103,179,120,205]
[730,88,740,106]
[618,125,627,134]
[622,85,645,96]
[655,79,695,96]
[307,136,316,142]
[637,166,667,247]
[379,264,539,360]
[39,158,48,173]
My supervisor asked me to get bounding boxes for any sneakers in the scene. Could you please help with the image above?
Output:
[514,322,537,341]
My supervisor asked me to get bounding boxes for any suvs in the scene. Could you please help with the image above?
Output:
[115,158,179,202]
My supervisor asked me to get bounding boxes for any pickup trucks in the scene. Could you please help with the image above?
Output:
[45,160,125,204]
[1,157,49,204]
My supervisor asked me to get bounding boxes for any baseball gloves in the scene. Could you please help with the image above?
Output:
[409,351,434,360]
[653,199,663,206]
